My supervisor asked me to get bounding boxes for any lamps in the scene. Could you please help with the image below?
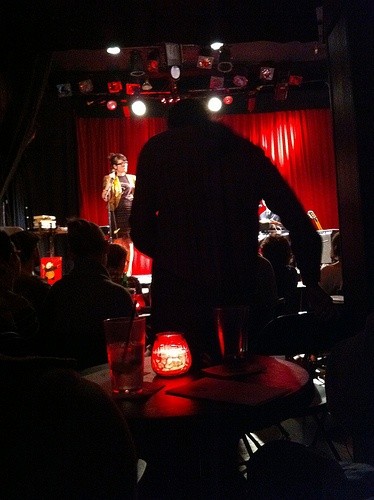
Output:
[128,50,145,76]
[38,256,64,283]
[216,47,233,73]
[144,50,160,72]
[151,332,193,377]
[232,67,250,87]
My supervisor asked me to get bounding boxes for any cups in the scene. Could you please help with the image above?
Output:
[103,318,145,393]
[131,294,146,310]
[151,332,191,377]
[153,377,191,415]
[215,306,249,369]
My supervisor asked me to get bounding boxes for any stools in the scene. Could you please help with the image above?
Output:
[305,377,341,463]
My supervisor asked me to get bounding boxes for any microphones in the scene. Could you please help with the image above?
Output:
[111,169,115,182]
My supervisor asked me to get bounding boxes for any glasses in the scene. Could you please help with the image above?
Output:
[116,162,128,165]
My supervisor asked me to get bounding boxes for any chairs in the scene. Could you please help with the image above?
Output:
[7,355,139,497]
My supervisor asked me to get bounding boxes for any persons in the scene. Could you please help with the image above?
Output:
[41,217,137,375]
[102,152,137,239]
[9,232,50,328]
[321,231,346,296]
[128,98,322,360]
[258,234,300,296]
[0,230,47,357]
[106,243,142,296]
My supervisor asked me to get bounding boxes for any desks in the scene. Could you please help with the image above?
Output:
[79,357,313,429]
